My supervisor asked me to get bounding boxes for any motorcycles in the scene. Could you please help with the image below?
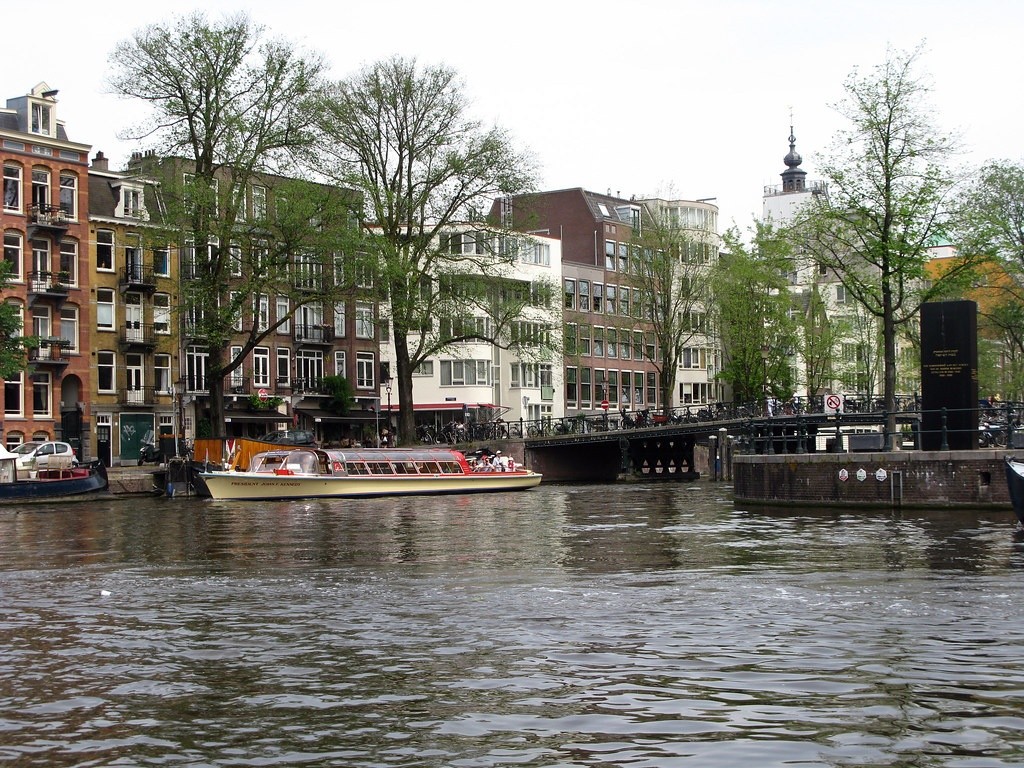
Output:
[137,430,161,466]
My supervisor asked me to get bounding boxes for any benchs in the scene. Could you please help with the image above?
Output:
[37,454,72,479]
[848,434,902,454]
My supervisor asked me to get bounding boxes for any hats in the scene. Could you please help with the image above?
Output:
[482,455,487,460]
[496,450,502,455]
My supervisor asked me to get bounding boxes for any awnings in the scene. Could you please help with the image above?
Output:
[293,408,387,423]
[379,405,513,412]
[222,409,295,422]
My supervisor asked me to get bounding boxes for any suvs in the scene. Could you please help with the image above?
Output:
[260,428,319,449]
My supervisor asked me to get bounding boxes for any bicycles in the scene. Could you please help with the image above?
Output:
[418,396,1024,447]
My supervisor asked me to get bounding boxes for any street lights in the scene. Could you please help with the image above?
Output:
[600,377,609,431]
[172,376,185,461]
[760,346,770,417]
[383,375,395,447]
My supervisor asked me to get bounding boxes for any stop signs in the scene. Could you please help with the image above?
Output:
[601,401,609,411]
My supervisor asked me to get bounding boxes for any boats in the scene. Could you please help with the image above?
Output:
[0,459,109,497]
[199,446,543,502]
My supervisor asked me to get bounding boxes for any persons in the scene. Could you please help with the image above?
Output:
[470,450,507,474]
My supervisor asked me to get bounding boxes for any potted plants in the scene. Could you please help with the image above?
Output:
[29,458,37,478]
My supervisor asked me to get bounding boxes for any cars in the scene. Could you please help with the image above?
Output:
[9,443,80,475]
